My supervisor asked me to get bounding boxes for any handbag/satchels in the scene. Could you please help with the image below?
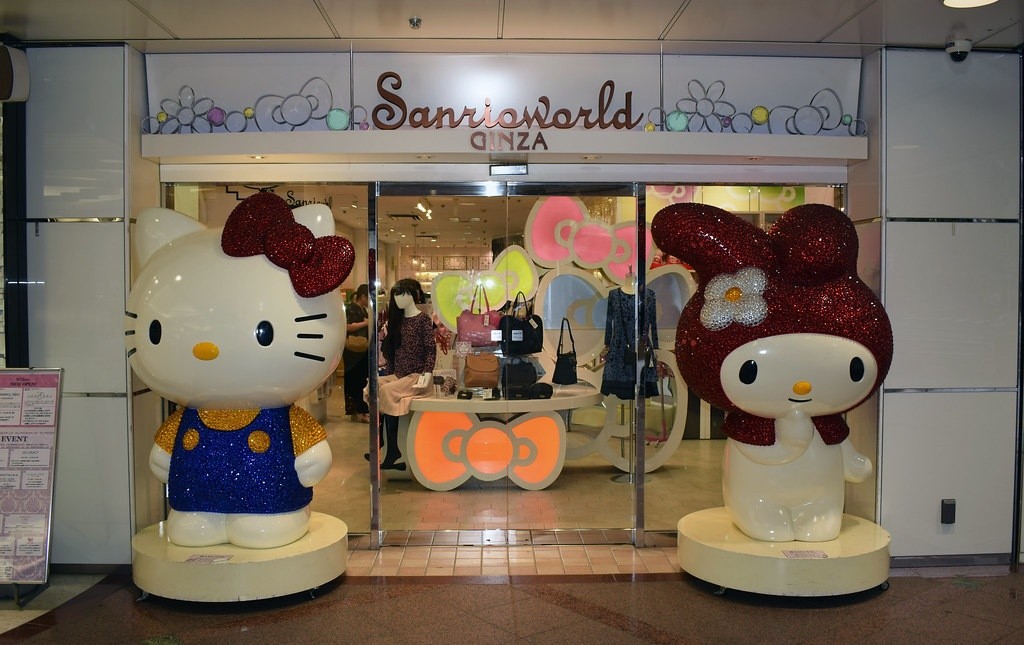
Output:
[464,354,500,388]
[625,345,645,366]
[502,357,537,387]
[499,291,543,356]
[345,335,368,352]
[456,284,502,347]
[552,318,577,384]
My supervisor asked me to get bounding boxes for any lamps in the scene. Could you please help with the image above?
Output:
[416,196,432,220]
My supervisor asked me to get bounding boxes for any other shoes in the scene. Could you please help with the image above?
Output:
[379,462,406,471]
[364,453,370,461]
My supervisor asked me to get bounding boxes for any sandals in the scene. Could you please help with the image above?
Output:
[351,414,370,423]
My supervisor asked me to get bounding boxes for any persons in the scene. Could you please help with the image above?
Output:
[600,273,659,401]
[364,279,437,474]
[342,283,388,424]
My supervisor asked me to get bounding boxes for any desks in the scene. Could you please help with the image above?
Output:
[373,385,604,491]
